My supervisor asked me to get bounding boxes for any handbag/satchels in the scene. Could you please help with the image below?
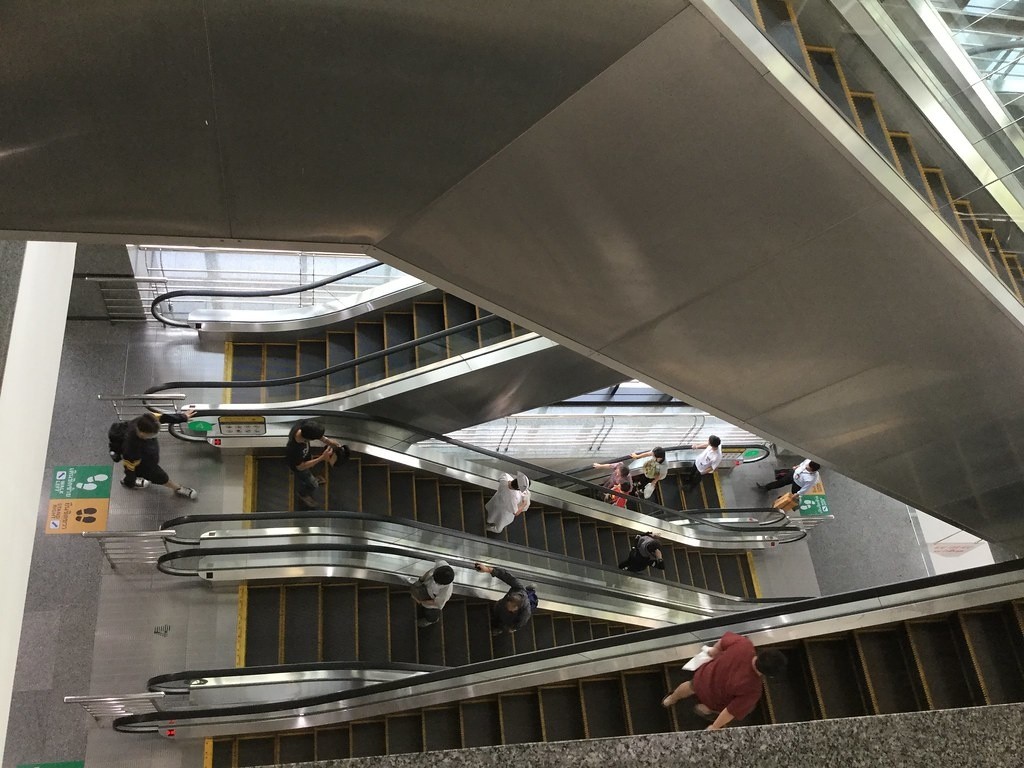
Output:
[775,469,793,480]
[412,582,433,601]
[644,483,655,499]
[773,492,800,512]
[612,497,627,509]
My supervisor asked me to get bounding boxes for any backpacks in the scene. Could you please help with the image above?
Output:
[109,421,128,462]
[643,459,655,478]
[510,588,538,611]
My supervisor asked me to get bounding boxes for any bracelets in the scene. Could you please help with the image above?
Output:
[322,437,328,443]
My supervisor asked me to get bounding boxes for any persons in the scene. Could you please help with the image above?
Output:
[756,459,820,501]
[593,462,633,508]
[120,409,197,499]
[477,563,531,637]
[629,446,668,493]
[412,559,455,627]
[485,471,531,533]
[285,418,341,508]
[659,631,786,730]
[619,531,665,572]
[682,436,723,492]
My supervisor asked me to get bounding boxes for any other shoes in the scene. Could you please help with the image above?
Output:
[682,484,691,491]
[662,690,674,707]
[310,475,319,484]
[622,567,628,570]
[693,705,719,721]
[416,617,439,627]
[635,489,644,495]
[486,524,492,531]
[632,482,641,486]
[298,494,319,507]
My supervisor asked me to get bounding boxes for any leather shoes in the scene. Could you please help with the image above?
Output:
[756,481,767,492]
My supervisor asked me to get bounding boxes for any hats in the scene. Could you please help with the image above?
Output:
[517,471,529,492]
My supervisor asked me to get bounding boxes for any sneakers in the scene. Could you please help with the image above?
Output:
[120,477,150,489]
[175,485,197,499]
